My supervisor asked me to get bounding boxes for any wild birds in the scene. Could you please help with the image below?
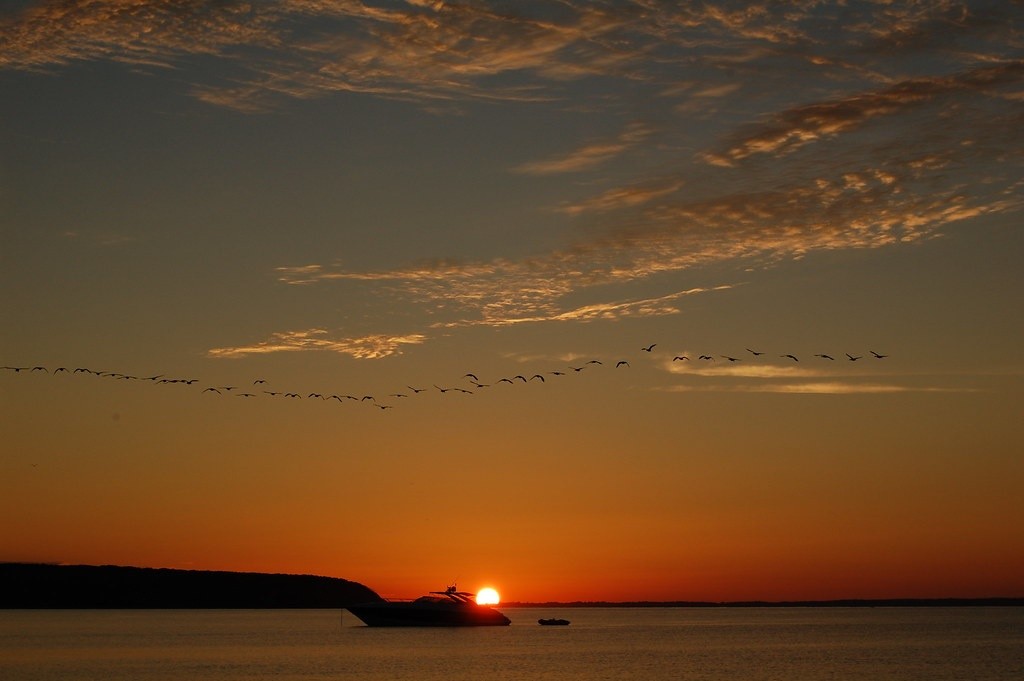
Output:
[495,378,513,384]
[747,349,765,355]
[550,372,564,375]
[585,360,601,364]
[846,353,863,361]
[673,356,689,361]
[617,361,630,367]
[869,351,887,358]
[464,374,478,380]
[512,375,526,382]
[4,366,452,410]
[781,354,799,361]
[721,355,741,362]
[699,355,715,362]
[455,388,473,395]
[814,354,834,360]
[569,366,586,371]
[470,381,490,388]
[642,344,656,352]
[529,374,544,383]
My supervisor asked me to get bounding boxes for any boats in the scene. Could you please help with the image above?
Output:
[346,582,512,627]
[538,617,570,625]
